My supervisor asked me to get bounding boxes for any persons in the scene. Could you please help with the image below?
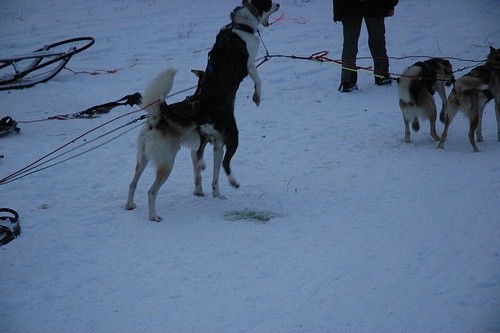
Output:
[333,0,399,93]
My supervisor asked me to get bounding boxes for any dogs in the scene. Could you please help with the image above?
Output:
[125,0,280,222]
[398,45,500,152]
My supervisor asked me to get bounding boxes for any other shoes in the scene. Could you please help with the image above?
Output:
[375,77,393,85]
[339,80,357,92]
